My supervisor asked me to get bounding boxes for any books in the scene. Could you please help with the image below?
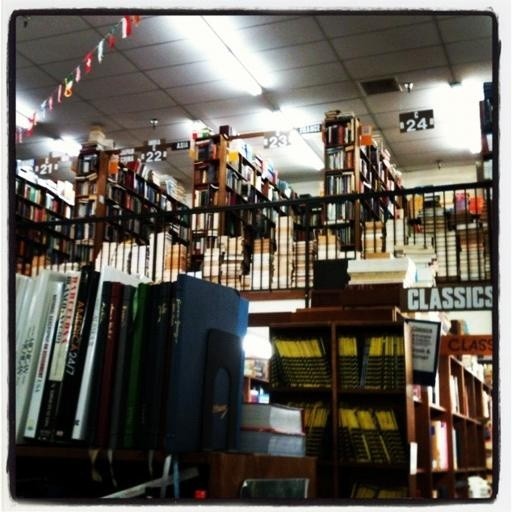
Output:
[15,108,493,499]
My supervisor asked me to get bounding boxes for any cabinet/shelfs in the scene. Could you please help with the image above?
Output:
[244,303,492,499]
[14,438,318,501]
[187,125,315,277]
[67,142,191,266]
[14,175,74,278]
[320,109,411,252]
